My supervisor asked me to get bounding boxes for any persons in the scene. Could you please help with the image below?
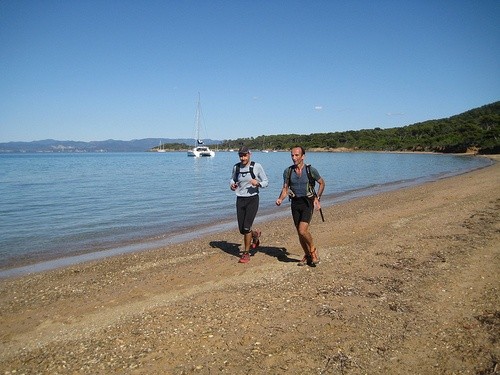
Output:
[275,145,325,267]
[229,147,269,263]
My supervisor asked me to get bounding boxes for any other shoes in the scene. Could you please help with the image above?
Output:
[298,253,312,266]
[251,229,261,249]
[239,254,250,263]
[309,248,321,265]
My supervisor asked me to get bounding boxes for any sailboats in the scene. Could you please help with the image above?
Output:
[157,141,166,153]
[186,91,216,157]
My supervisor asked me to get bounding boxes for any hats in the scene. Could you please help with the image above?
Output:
[238,147,249,153]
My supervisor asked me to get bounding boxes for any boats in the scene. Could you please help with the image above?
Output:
[273,148,277,152]
[262,150,268,153]
